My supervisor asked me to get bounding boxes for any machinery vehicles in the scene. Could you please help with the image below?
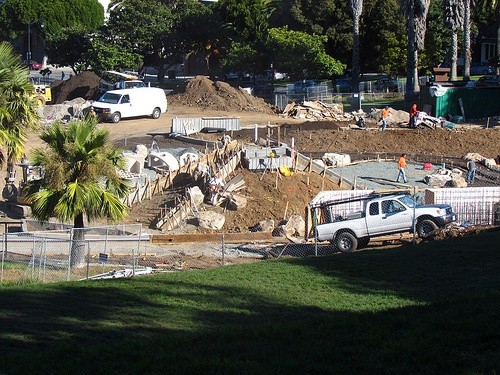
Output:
[22,74,52,106]
[2,160,45,220]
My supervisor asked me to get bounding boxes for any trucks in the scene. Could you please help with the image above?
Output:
[95,78,147,93]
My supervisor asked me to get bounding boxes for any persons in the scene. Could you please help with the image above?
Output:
[89,107,100,130]
[61,71,65,80]
[410,103,420,129]
[466,159,475,185]
[397,153,409,183]
[386,202,398,213]
[378,106,394,131]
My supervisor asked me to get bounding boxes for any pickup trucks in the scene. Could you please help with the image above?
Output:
[314,192,457,253]
[21,59,42,70]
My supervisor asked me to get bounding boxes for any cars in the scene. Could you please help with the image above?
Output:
[293,79,320,93]
[222,72,238,82]
[461,61,499,76]
[336,78,353,93]
[373,77,398,92]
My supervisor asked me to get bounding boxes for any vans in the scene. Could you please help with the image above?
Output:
[91,86,168,123]
